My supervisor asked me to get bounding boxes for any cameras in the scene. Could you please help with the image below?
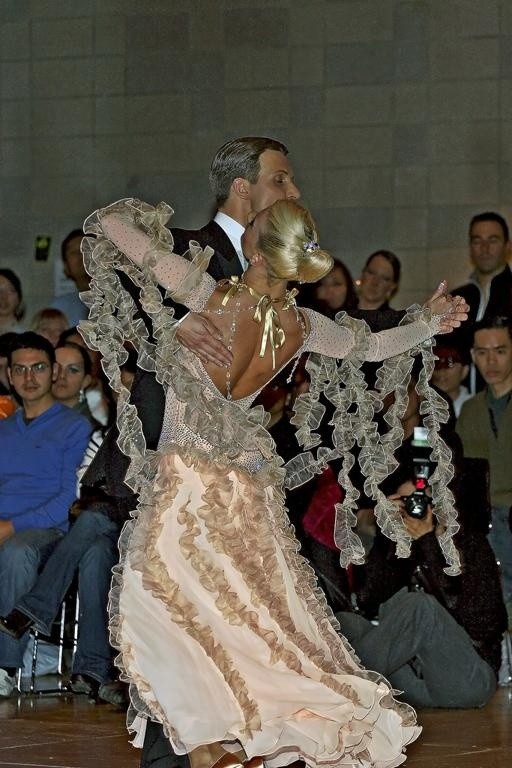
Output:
[398,486,432,518]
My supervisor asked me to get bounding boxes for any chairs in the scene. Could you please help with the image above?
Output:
[13,602,82,708]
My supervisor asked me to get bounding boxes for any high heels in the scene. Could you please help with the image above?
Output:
[0,608,35,639]
[61,673,101,704]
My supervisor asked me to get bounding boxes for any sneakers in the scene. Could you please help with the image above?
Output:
[0,668,16,697]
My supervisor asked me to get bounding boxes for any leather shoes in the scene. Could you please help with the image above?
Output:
[210,752,244,768]
[244,757,264,768]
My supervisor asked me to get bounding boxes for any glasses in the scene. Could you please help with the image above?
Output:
[12,364,51,376]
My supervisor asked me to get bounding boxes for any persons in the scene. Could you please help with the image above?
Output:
[75,138,512,768]
[0,228,146,706]
